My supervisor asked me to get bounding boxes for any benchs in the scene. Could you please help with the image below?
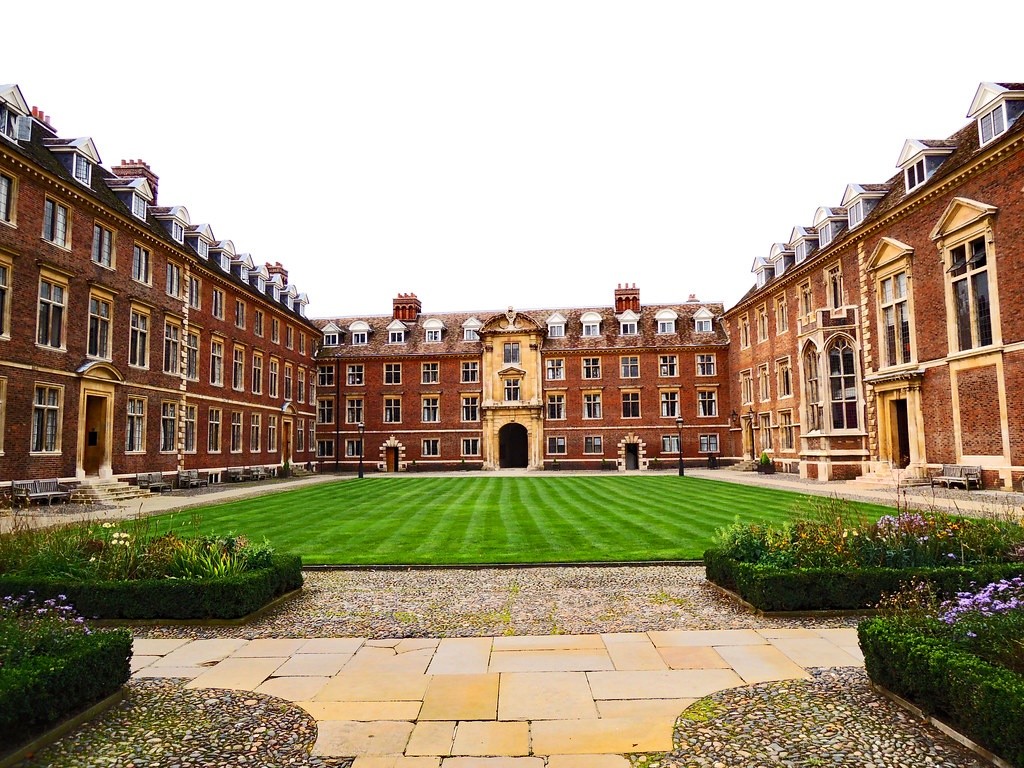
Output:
[227,465,252,482]
[178,469,209,488]
[8,478,72,506]
[929,463,984,492]
[249,466,273,481]
[135,471,174,493]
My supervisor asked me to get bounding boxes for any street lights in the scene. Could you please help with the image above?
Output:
[747,405,756,463]
[357,422,366,478]
[675,414,684,476]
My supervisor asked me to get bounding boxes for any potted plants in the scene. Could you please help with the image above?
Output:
[755,453,776,474]
